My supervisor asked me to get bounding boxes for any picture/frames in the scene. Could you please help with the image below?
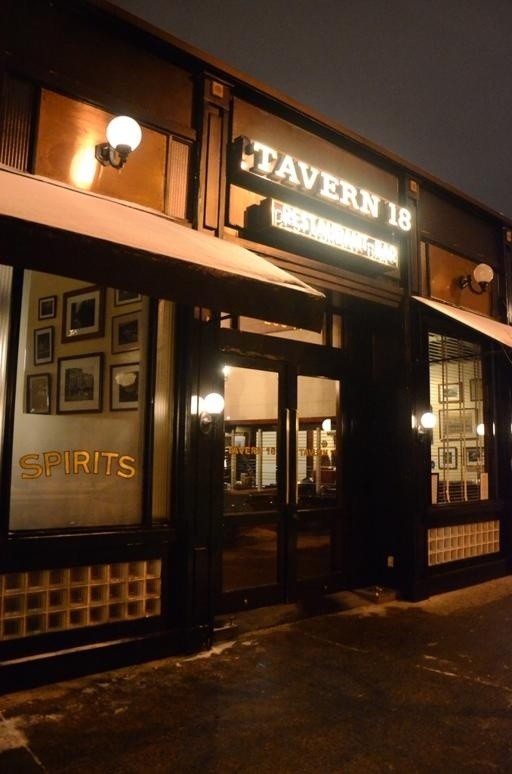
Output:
[27,284,141,417]
[438,379,485,470]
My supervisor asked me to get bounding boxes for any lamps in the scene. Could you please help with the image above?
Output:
[198,393,226,442]
[457,264,494,296]
[95,113,142,171]
[416,412,436,446]
